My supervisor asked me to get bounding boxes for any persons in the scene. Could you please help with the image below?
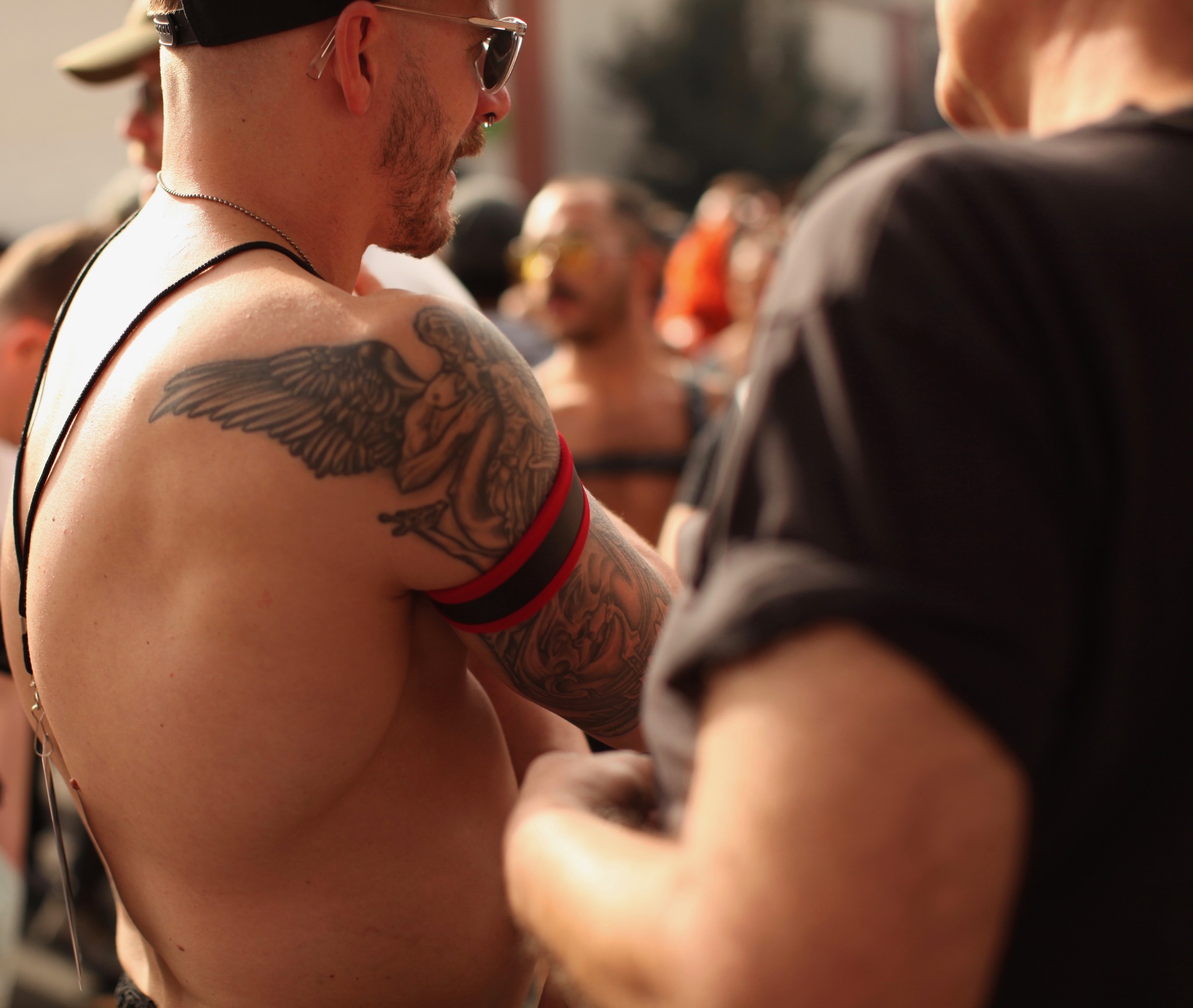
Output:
[0,0,1192,1007]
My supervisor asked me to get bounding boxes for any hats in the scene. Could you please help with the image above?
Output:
[55,0,157,83]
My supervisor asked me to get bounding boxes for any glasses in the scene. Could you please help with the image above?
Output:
[306,2,527,94]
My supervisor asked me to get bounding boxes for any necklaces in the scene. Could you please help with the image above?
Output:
[157,167,315,269]
